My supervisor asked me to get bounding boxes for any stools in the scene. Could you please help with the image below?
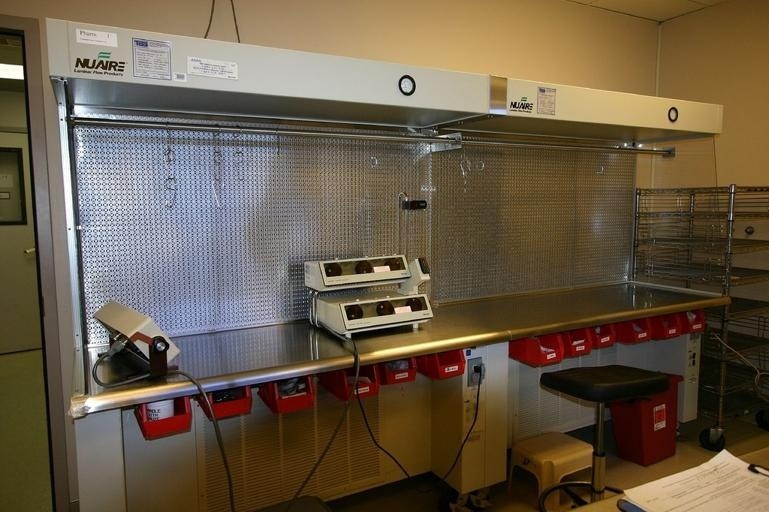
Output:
[539,365,674,512]
[505,430,595,511]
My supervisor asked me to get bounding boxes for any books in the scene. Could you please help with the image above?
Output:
[613,449,769,512]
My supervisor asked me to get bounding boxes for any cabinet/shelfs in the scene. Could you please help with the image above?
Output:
[630,183,769,452]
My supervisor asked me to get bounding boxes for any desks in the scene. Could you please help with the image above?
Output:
[566,446,769,512]
[68,276,733,512]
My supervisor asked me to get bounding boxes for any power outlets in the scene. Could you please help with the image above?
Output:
[466,356,484,389]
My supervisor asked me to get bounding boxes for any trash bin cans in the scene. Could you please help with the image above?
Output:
[609,374,683,465]
